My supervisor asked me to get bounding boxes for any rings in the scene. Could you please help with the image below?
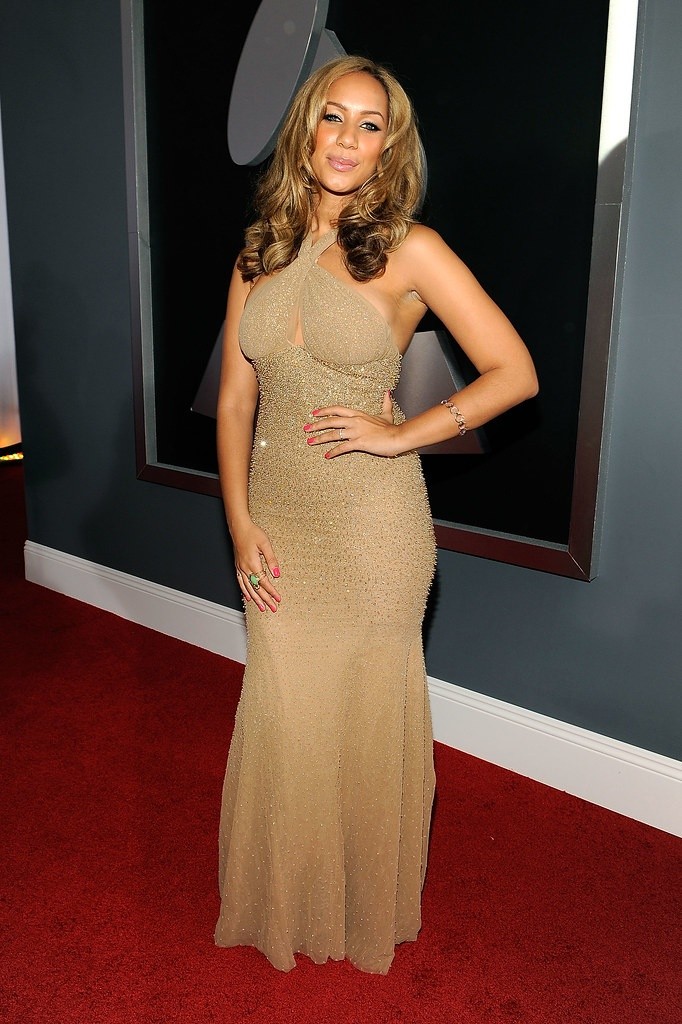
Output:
[237,573,242,577]
[249,570,266,589]
[340,429,343,441]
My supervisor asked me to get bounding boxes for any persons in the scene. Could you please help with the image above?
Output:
[215,58,540,977]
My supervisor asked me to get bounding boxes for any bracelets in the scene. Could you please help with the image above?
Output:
[440,398,468,437]
